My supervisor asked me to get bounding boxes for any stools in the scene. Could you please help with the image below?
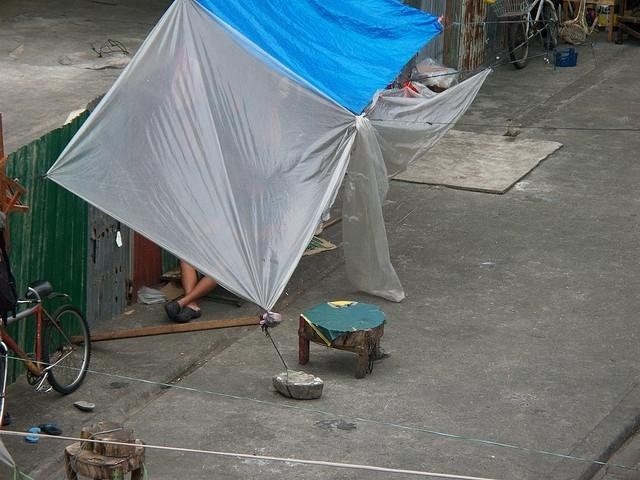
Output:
[298,300,387,378]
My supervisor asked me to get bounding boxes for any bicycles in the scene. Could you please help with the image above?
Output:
[0,280,91,429]
[488,0,559,70]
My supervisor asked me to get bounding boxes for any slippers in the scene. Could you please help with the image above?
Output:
[175,306,202,322]
[3,400,95,442]
[165,298,182,319]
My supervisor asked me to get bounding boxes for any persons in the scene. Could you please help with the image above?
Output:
[159,258,220,321]
